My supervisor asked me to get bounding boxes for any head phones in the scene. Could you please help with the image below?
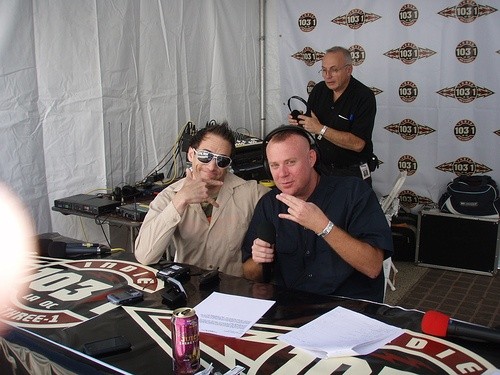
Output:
[288,96,311,127]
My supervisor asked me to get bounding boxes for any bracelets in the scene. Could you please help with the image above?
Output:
[317,125,328,140]
[318,221,334,239]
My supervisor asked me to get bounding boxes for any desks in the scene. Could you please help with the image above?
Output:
[0,230,500,375]
[52,208,147,257]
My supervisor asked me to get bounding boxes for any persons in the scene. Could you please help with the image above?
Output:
[135,123,273,276]
[288,45,376,187]
[242,125,393,305]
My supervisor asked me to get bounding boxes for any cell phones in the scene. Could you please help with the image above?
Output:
[83,335,132,358]
[107,288,144,305]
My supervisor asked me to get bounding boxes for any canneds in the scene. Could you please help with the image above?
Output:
[171,308,200,375]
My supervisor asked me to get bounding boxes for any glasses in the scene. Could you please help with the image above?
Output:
[194,148,232,170]
[318,64,348,77]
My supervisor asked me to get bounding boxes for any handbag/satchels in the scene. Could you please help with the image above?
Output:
[437,175,499,216]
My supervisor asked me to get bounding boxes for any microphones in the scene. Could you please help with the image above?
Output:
[47,242,111,258]
[421,309,489,339]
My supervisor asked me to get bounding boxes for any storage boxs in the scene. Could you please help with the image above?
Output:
[415,210,500,276]
